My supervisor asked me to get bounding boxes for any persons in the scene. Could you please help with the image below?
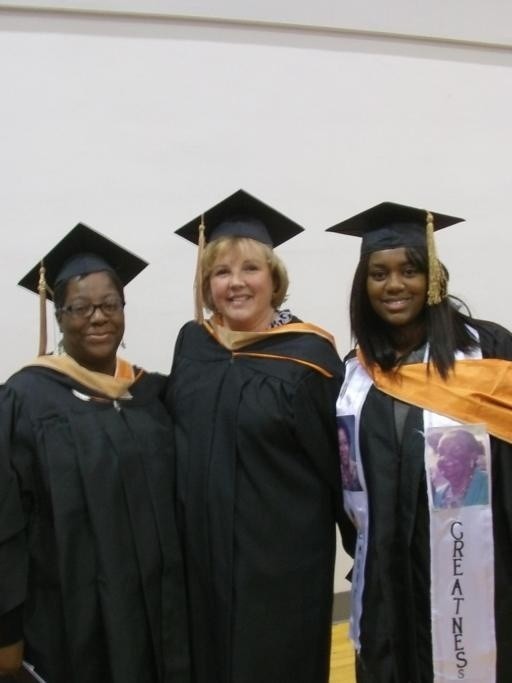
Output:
[170,189,357,683]
[432,430,488,509]
[0,224,171,683]
[337,417,362,491]
[325,202,512,683]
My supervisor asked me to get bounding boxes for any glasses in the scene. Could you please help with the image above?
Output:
[54,295,125,321]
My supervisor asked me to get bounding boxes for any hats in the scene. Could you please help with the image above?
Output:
[325,201,466,306]
[18,223,149,356]
[174,189,306,326]
